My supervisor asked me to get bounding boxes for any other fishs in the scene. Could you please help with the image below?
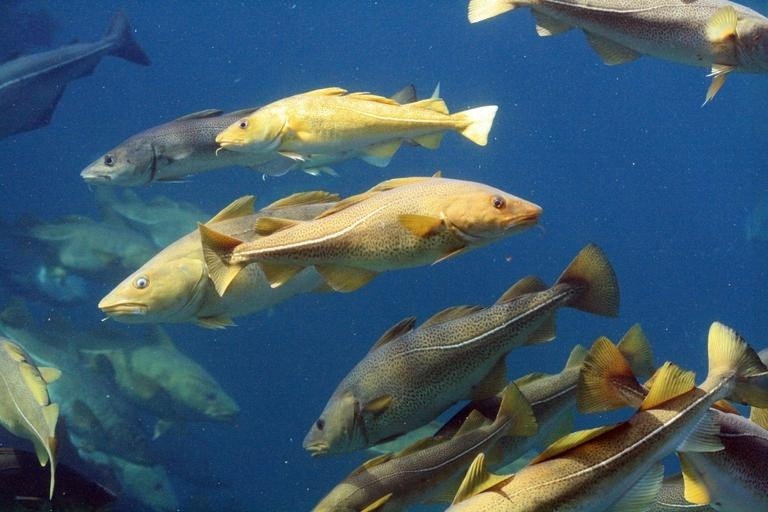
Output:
[0,189,240,512]
